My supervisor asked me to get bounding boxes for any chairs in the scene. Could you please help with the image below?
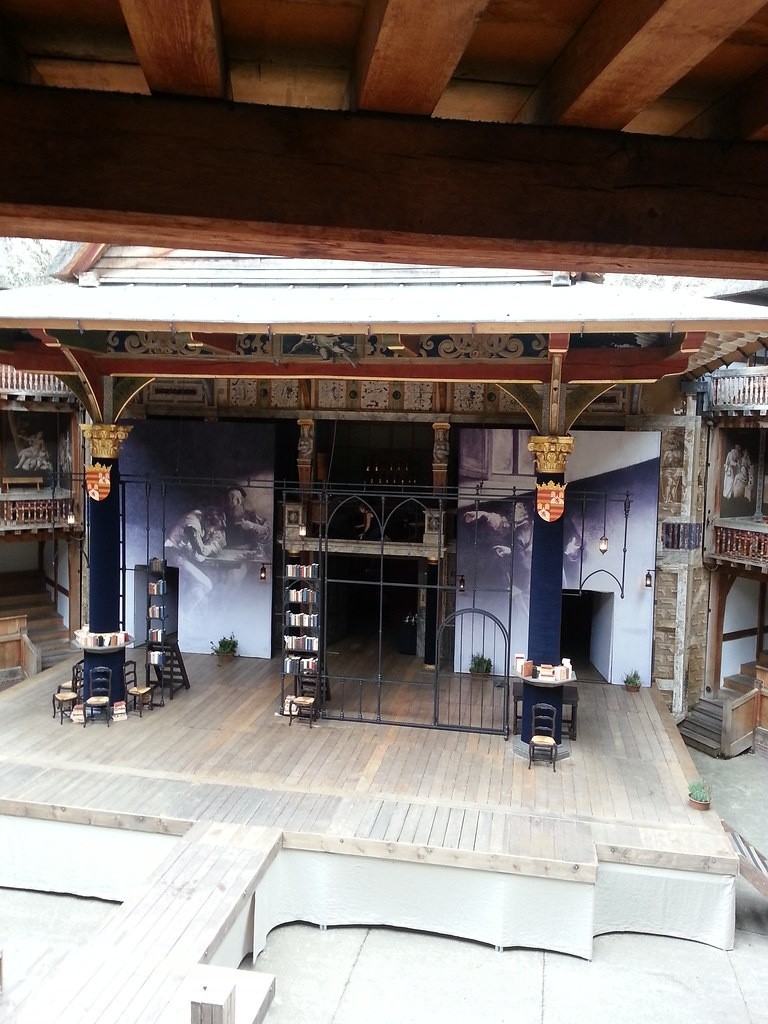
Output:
[528,703,557,773]
[289,671,320,728]
[56,658,84,710]
[84,666,112,728]
[122,660,153,718]
[52,666,82,724]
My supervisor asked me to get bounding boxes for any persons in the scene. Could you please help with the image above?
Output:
[213,481,271,609]
[354,501,390,542]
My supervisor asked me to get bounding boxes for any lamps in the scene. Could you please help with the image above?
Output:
[67,510,76,533]
[260,563,272,580]
[599,489,608,554]
[644,568,661,587]
[299,523,307,542]
[455,575,466,592]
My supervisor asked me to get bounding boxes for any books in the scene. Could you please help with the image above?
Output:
[536,658,572,680]
[512,653,534,677]
[147,650,166,665]
[143,557,167,572]
[284,656,318,674]
[70,703,87,722]
[286,563,319,578]
[148,605,165,619]
[283,694,300,715]
[148,628,164,642]
[285,635,319,651]
[285,611,318,627]
[289,588,319,603]
[73,624,129,647]
[111,701,127,722]
[147,580,166,595]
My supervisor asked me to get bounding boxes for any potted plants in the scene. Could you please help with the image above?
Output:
[468,653,492,680]
[210,633,238,666]
[688,778,712,810]
[620,668,642,693]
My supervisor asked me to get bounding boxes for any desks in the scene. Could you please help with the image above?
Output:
[513,681,580,741]
